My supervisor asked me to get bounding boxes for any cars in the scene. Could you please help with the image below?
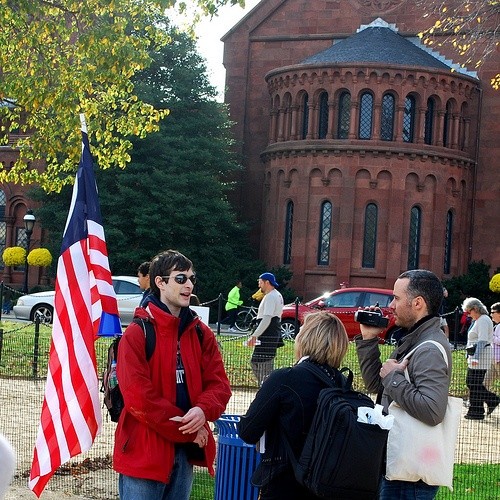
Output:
[280,288,397,342]
[15,277,145,327]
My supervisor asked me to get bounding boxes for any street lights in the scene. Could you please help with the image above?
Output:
[22,209,36,295]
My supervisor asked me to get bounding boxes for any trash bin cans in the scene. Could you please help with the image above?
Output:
[212,415,261,500]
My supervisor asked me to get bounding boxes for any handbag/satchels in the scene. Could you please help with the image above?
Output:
[385,396,463,492]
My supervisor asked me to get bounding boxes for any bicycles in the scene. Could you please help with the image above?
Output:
[234,299,260,332]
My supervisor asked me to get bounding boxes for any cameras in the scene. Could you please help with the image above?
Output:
[354,309,389,329]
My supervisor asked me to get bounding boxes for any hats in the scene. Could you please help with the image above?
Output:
[259,273,279,286]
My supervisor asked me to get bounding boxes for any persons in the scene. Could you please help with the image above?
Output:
[355,268,453,500]
[236,311,356,500]
[137,261,151,308]
[461,296,500,420]
[339,282,349,289]
[489,302,500,376]
[248,272,284,389]
[112,249,232,500]
[2,285,13,316]
[216,280,243,331]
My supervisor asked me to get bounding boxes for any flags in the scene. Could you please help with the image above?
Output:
[28,131,123,497]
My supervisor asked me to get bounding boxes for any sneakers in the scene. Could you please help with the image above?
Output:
[216,322,222,329]
[228,327,236,332]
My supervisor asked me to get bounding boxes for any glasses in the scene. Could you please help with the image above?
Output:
[467,308,473,315]
[162,274,196,284]
[491,312,497,314]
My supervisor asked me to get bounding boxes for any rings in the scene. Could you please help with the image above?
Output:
[202,438,206,442]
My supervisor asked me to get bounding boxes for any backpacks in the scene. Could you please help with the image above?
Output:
[278,363,389,500]
[100,316,204,423]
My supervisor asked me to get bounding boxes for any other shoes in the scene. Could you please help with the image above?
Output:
[487,399,500,415]
[464,414,485,420]
